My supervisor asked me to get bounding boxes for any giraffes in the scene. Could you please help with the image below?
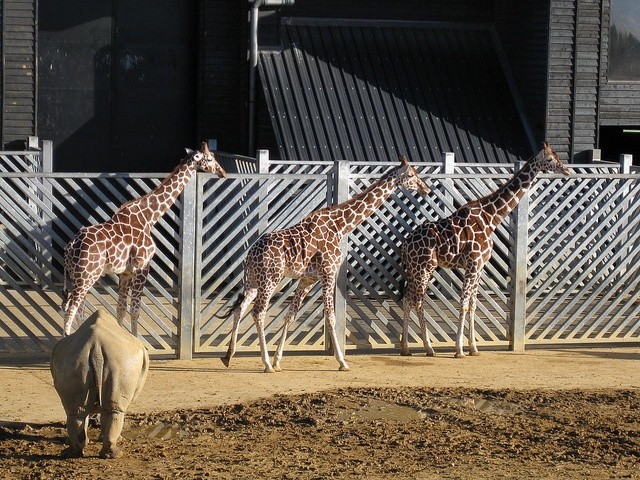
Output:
[61,142,228,340]
[395,141,572,358]
[212,154,431,373]
